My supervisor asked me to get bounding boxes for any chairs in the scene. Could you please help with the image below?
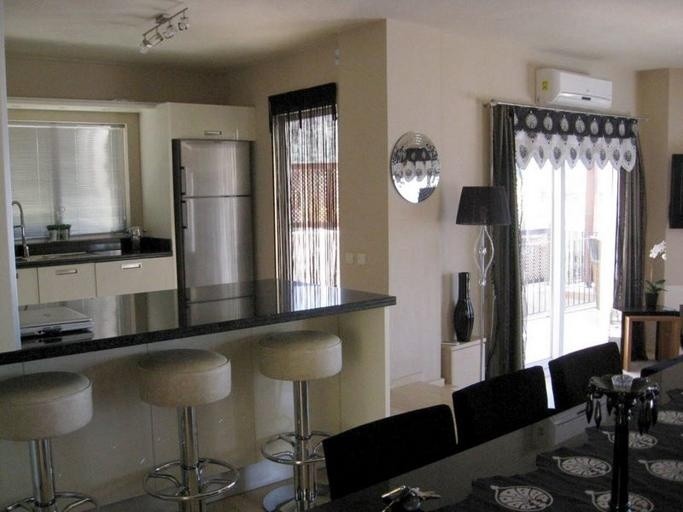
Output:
[142,349,241,507]
[548,342,623,413]
[584,237,598,304]
[452,364,550,450]
[319,401,459,500]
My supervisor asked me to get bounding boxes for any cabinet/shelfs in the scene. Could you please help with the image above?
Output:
[35,261,99,306]
[608,306,680,372]
[97,257,179,299]
[140,101,256,142]
[13,269,38,307]
[440,339,486,393]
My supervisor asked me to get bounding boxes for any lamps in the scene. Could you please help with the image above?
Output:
[456,184,512,381]
[138,7,194,55]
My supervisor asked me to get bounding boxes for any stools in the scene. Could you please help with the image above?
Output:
[251,331,345,507]
[1,371,104,511]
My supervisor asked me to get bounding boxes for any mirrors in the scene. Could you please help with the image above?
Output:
[390,130,440,206]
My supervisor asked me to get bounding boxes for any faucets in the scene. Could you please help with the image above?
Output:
[12,200,30,257]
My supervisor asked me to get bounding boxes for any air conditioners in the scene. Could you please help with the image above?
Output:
[534,69,615,112]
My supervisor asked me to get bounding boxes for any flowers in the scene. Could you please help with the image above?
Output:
[642,241,668,295]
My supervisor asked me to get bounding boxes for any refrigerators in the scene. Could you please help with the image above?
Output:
[173,138,256,290]
[179,281,256,327]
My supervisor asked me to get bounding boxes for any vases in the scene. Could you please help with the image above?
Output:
[644,292,658,308]
[452,272,474,341]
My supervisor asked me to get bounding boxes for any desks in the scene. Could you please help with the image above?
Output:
[0,278,399,512]
[300,363,681,512]
